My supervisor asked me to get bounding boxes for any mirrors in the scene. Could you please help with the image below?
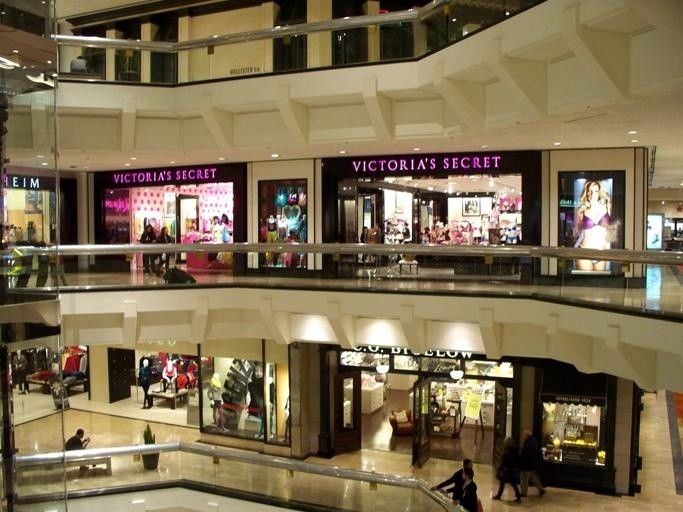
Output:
[176,194,199,263]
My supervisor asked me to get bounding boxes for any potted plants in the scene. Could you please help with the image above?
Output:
[142,423,160,470]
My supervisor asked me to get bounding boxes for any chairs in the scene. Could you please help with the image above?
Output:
[389,407,413,441]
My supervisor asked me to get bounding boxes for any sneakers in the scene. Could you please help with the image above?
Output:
[491,490,546,502]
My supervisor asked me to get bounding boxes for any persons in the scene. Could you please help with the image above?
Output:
[488,203,500,230]
[464,200,477,213]
[438,468,478,512]
[489,435,522,504]
[160,360,178,392]
[69,55,91,75]
[60,428,96,472]
[357,226,370,263]
[518,429,545,498]
[294,214,306,268]
[572,177,613,274]
[190,212,232,245]
[154,226,172,278]
[505,221,518,245]
[139,224,157,274]
[45,234,68,287]
[255,401,275,442]
[17,354,30,394]
[137,358,155,409]
[420,226,432,246]
[264,214,279,267]
[429,459,472,508]
[11,354,23,394]
[399,221,410,244]
[206,373,226,433]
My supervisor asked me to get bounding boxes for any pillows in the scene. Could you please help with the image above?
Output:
[393,410,408,423]
